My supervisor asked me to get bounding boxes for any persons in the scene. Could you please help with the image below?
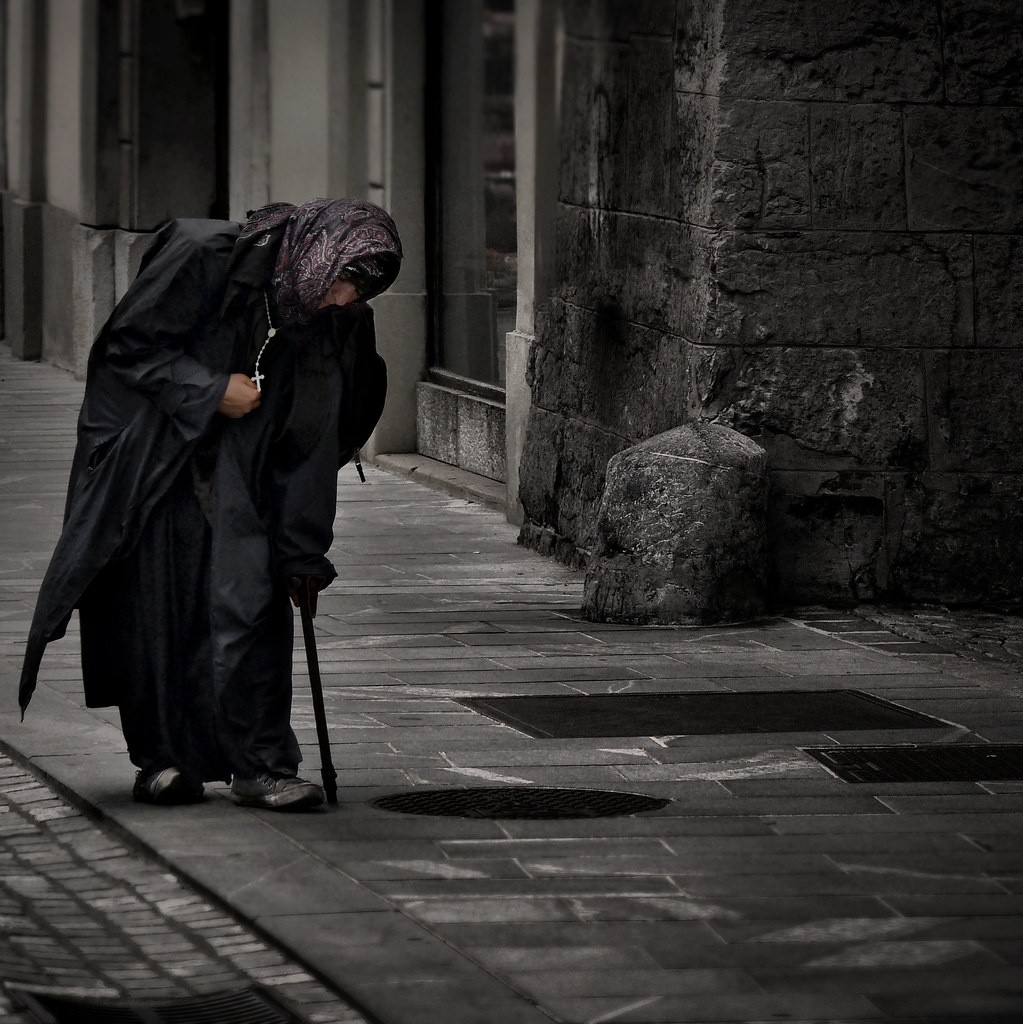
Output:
[15,199,402,813]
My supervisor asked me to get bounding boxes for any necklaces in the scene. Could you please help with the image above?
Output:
[249,288,285,392]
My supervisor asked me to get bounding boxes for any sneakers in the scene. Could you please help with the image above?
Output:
[133,766,205,804]
[230,768,324,813]
